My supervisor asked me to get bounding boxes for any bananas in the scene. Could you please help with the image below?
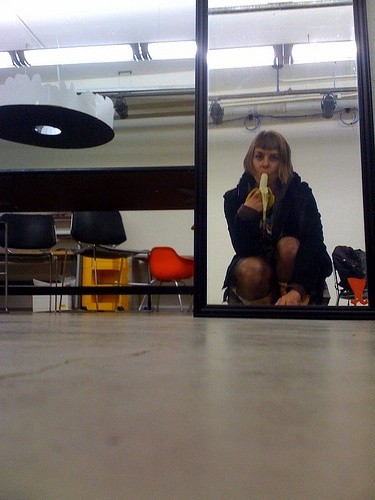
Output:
[245,172,276,225]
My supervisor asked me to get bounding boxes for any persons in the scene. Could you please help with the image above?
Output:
[221,130,333,307]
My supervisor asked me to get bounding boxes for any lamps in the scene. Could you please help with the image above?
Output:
[114,97,128,119]
[207,98,224,125]
[323,92,337,118]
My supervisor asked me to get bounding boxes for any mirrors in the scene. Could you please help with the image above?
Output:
[192,1,374,322]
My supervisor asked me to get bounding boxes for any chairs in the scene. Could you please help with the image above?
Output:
[0,211,59,312]
[333,249,367,307]
[137,247,194,313]
[60,209,152,311]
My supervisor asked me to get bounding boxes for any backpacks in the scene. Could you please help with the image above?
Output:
[333,244,368,296]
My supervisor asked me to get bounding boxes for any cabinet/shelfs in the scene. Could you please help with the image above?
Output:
[80,254,128,311]
